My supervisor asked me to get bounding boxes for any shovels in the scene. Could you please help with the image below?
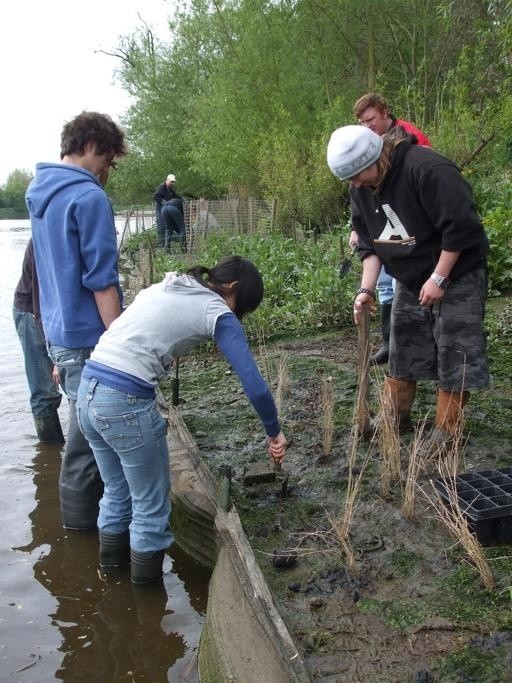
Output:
[339,243,357,280]
[269,439,289,499]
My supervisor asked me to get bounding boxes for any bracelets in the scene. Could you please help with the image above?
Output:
[357,288,377,302]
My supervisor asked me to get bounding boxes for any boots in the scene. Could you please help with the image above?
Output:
[412,387,471,458]
[350,373,418,436]
[367,303,393,366]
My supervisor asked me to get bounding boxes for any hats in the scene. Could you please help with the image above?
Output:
[326,123,384,181]
[166,174,177,182]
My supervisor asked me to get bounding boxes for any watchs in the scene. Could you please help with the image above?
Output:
[431,272,451,290]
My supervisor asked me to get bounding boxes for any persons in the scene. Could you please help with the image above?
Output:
[12,236,66,445]
[347,92,434,367]
[326,125,493,462]
[74,254,291,584]
[22,111,129,534]
[160,197,197,254]
[152,172,182,247]
